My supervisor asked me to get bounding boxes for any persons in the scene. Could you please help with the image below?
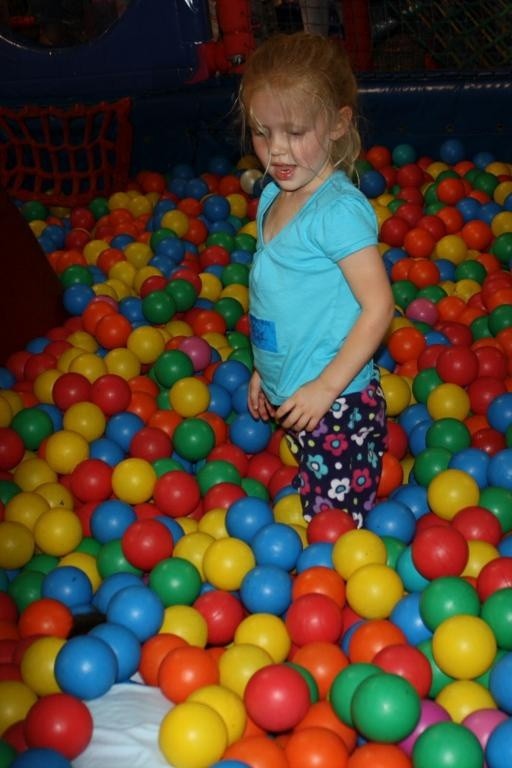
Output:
[238,29,396,532]
